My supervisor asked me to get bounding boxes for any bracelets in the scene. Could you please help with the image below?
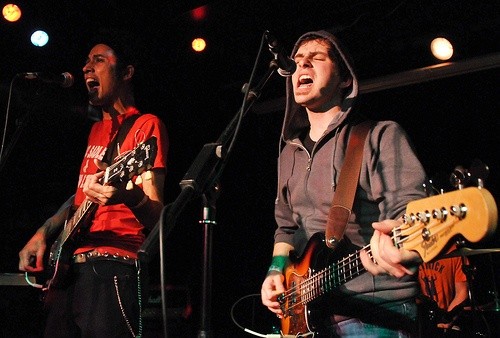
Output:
[267,255,290,272]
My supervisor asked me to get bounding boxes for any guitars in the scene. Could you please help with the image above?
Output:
[29,137,157,290]
[273,188,497,338]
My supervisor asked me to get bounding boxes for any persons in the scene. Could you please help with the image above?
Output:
[261,31,437,338]
[419,243,476,330]
[19,33,168,338]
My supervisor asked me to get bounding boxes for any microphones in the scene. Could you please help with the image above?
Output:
[266,30,297,77]
[22,72,73,87]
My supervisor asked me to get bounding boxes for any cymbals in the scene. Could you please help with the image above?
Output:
[425,245,500,263]
